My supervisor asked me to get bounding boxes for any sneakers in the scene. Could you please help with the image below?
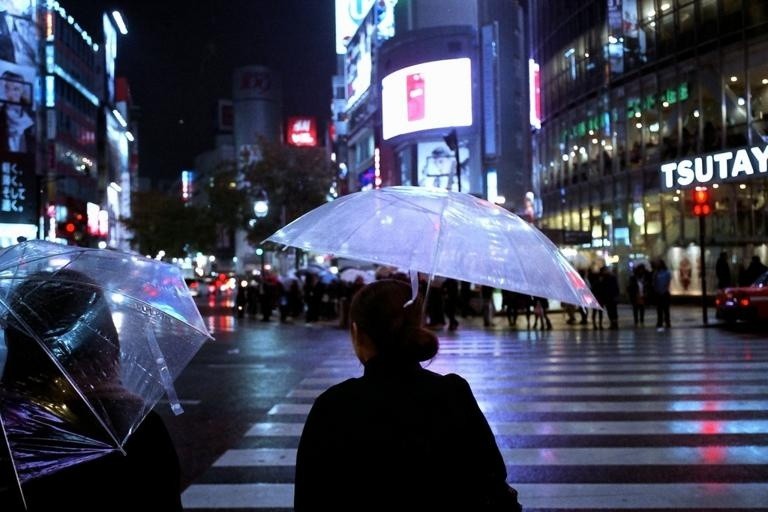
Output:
[426,313,673,334]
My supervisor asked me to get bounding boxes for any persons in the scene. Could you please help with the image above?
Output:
[291,277,522,510]
[1,268,185,511]
[23,24,44,67]
[1,72,31,154]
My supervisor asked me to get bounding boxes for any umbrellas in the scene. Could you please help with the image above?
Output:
[0,239,216,484]
[259,181,608,322]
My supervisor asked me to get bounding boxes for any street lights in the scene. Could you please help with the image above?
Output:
[253,190,269,279]
[442,126,461,193]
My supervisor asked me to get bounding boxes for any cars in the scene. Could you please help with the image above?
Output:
[100,268,237,311]
[715,270,768,332]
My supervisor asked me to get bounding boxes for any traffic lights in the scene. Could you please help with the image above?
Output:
[694,187,710,216]
[74,211,87,245]
[65,222,76,235]
